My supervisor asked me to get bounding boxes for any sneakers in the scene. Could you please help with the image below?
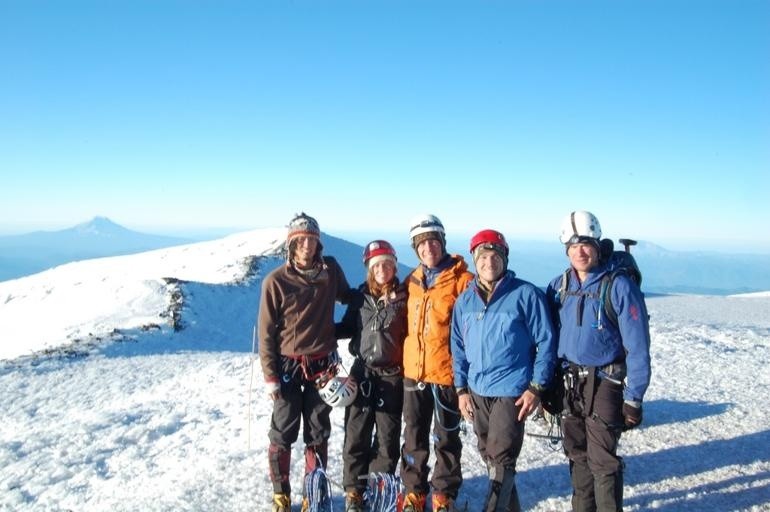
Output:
[402,490,427,512]
[300,496,311,512]
[271,493,291,512]
[344,487,363,512]
[432,493,456,511]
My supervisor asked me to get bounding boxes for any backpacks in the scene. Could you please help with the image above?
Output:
[555,249,649,319]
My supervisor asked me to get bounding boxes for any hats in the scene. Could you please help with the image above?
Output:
[368,255,399,273]
[286,215,323,251]
[472,244,508,274]
[412,232,446,262]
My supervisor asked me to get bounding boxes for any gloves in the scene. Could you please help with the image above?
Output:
[620,401,643,432]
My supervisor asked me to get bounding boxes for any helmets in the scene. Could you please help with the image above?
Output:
[362,240,397,263]
[468,229,510,254]
[314,376,359,408]
[559,210,603,243]
[409,214,446,239]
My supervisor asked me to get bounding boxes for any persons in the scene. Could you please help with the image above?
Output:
[451,229,557,512]
[258,216,358,512]
[542,210,652,512]
[401,212,477,511]
[333,240,408,512]
